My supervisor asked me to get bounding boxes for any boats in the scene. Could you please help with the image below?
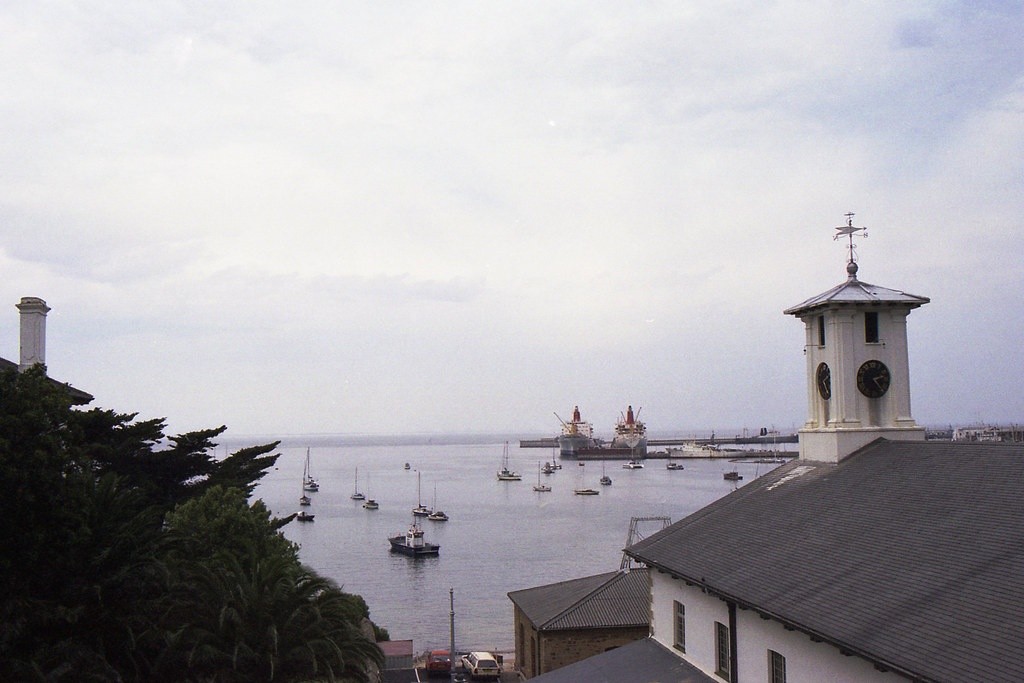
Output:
[297,511,316,521]
[623,458,644,469]
[723,469,743,481]
[609,405,648,455]
[665,459,684,470]
[553,406,602,456]
[665,433,748,458]
[387,512,441,558]
[573,487,600,495]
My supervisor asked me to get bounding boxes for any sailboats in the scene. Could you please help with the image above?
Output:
[350,465,380,510]
[532,448,562,491]
[599,447,612,486]
[412,471,450,521]
[299,446,320,506]
[496,439,523,479]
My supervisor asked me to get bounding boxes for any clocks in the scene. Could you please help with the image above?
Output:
[818,362,831,400]
[856,360,890,399]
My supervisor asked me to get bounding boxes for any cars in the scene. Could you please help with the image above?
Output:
[460,651,502,679]
[425,650,452,673]
